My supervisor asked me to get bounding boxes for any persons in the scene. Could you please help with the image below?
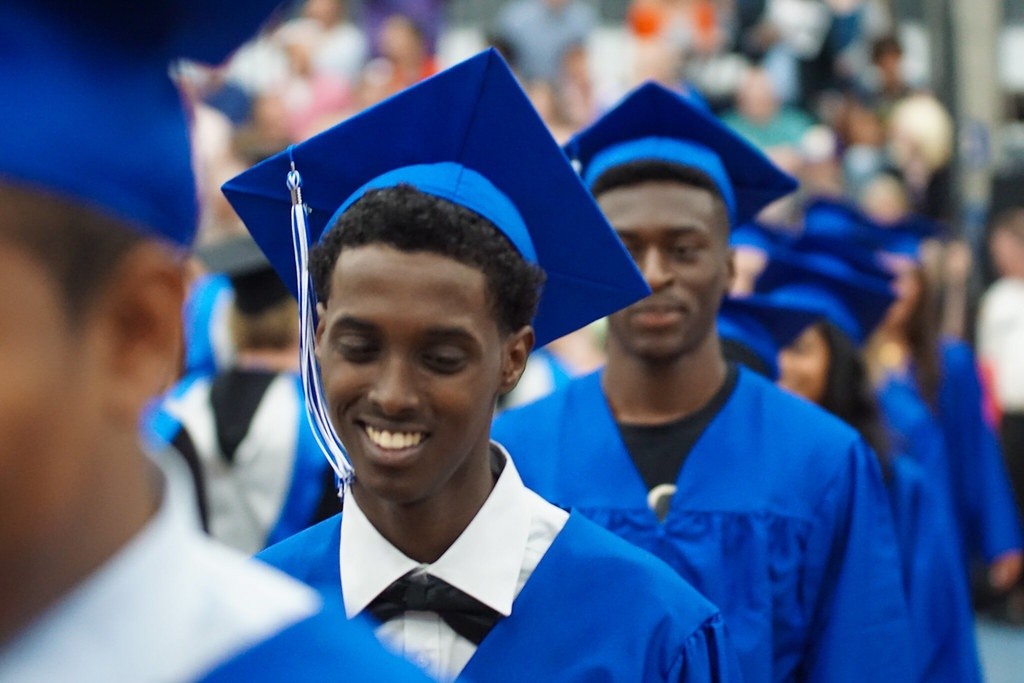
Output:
[171,0,1024,683]
[0,0,473,683]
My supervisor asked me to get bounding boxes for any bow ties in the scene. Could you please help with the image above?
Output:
[366,578,499,648]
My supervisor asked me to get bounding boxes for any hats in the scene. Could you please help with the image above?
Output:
[0,0,283,247]
[709,193,924,382]
[221,47,651,498]
[558,78,800,241]
